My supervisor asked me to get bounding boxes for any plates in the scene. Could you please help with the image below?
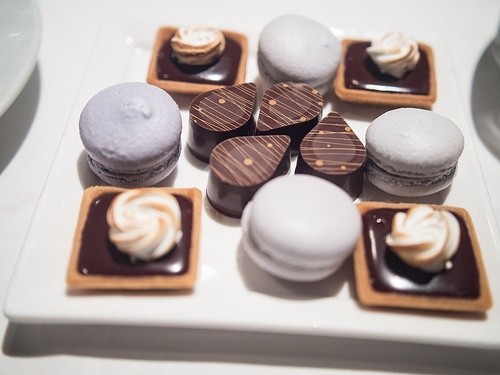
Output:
[2,15,500,351]
[0,0,44,118]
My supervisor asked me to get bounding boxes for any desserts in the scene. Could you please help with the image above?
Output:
[256,14,340,88]
[363,108,464,197]
[335,29,437,108]
[65,187,201,290]
[354,201,493,312]
[79,82,181,187]
[186,83,366,218]
[146,24,248,94]
[239,174,361,281]
